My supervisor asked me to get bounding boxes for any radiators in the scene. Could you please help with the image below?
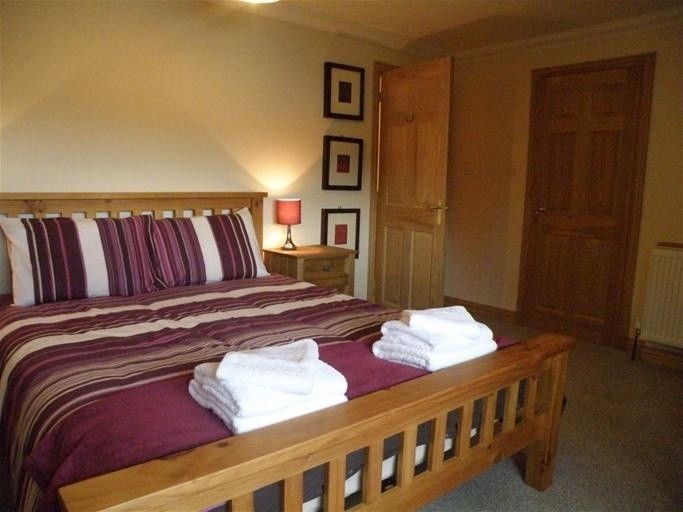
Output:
[627,244,681,363]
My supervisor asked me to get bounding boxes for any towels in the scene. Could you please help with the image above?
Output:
[364,316,498,371]
[398,302,481,337]
[184,361,348,434]
[216,336,323,393]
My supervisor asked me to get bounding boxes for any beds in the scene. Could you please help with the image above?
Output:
[1,185,578,511]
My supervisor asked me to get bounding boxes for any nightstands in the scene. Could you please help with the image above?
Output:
[261,242,359,296]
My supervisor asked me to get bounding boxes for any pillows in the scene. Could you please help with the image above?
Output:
[0,210,169,309]
[149,205,271,293]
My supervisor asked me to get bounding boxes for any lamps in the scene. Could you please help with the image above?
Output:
[274,197,302,250]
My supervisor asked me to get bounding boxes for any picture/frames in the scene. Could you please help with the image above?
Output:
[323,59,364,120]
[321,135,363,191]
[319,208,360,258]
